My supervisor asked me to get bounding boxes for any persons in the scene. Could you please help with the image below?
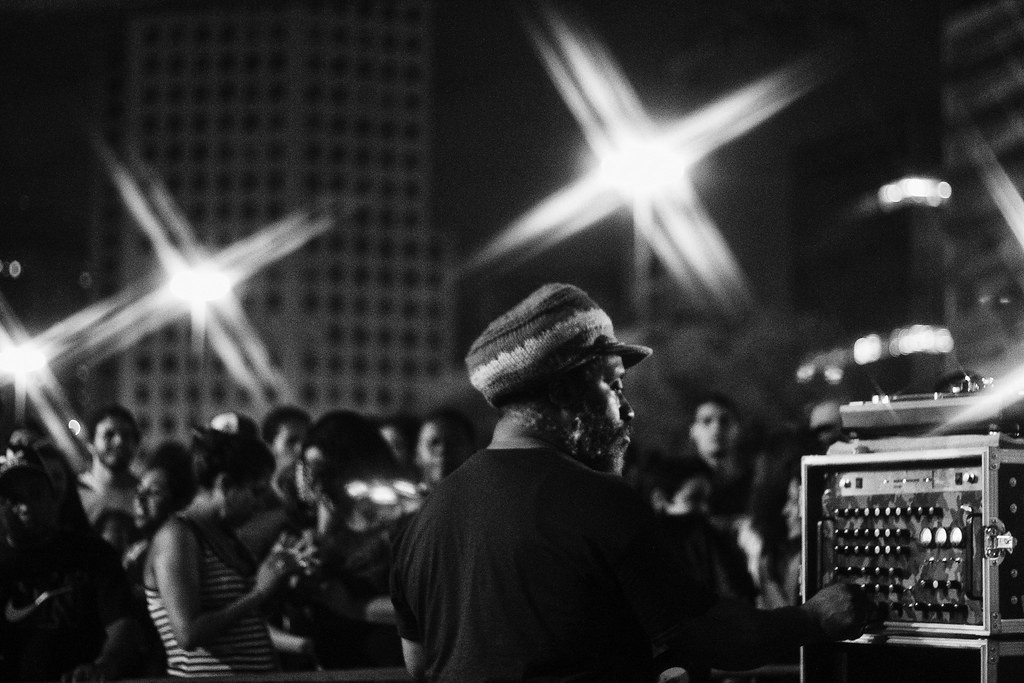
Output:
[389,284,868,683]
[649,393,807,608]
[0,400,479,682]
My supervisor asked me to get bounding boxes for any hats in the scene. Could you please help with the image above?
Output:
[464,281,655,411]
